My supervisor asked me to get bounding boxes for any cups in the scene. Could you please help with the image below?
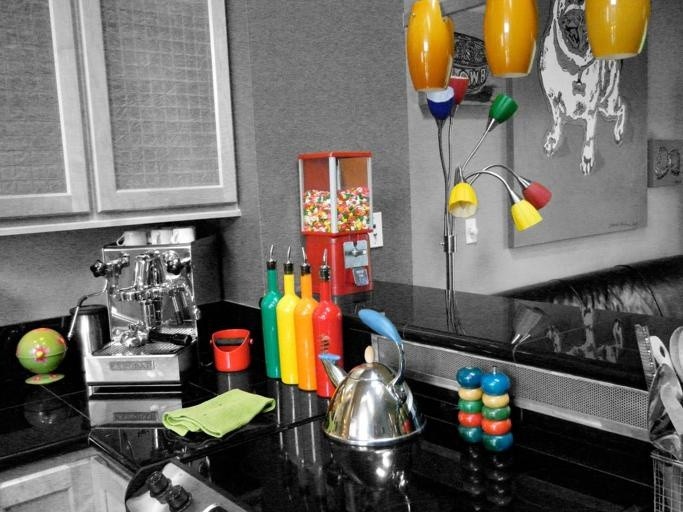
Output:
[116,227,195,246]
[71,305,107,404]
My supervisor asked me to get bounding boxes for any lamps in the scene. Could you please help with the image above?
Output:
[443,288,589,361]
[480,1,535,77]
[432,77,549,325]
[584,0,648,64]
[400,1,453,93]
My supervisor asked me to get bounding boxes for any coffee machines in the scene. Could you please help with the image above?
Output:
[84,235,221,398]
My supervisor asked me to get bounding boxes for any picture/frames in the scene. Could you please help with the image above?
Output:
[507,1,648,249]
[438,27,491,110]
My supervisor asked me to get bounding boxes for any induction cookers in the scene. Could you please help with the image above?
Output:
[121,417,652,512]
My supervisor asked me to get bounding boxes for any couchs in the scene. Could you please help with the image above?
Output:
[487,256,682,328]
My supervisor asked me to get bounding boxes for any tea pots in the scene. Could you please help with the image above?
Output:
[318,309,427,446]
[329,446,414,503]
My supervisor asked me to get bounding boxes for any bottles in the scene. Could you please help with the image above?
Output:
[262,245,343,398]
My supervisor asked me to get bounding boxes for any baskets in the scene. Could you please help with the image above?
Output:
[650,449,682,512]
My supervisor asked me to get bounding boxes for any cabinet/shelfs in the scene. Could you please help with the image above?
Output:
[0,1,241,238]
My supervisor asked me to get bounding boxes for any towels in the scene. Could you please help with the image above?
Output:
[162,388,276,440]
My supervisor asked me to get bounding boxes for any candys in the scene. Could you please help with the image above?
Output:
[303,185,370,232]
